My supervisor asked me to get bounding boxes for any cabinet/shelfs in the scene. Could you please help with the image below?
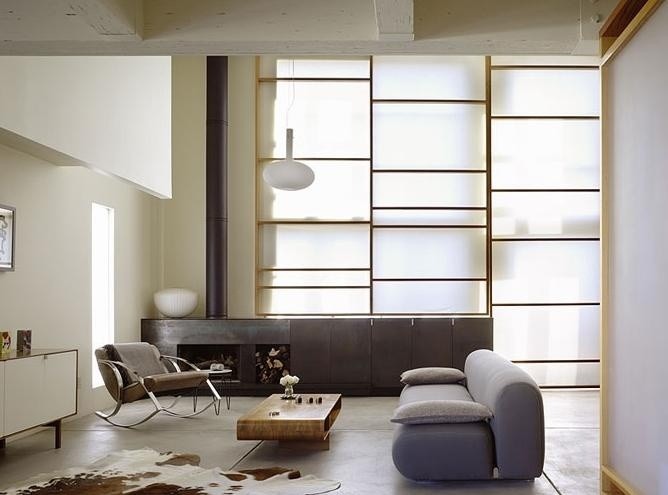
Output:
[0,346,79,449]
[137,315,290,398]
[371,316,452,399]
[288,316,370,397]
[452,318,492,374]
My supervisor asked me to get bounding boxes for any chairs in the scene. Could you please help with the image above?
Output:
[93,337,223,430]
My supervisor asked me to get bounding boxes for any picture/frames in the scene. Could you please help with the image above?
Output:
[0,202,17,273]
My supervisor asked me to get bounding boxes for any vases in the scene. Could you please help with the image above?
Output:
[283,384,294,399]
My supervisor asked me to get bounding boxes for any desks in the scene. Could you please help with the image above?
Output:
[191,367,233,416]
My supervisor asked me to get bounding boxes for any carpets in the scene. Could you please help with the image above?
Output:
[0,447,345,495]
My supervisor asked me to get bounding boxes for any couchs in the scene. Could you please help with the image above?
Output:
[391,345,547,482]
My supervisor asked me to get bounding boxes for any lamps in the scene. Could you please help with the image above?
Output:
[260,60,314,191]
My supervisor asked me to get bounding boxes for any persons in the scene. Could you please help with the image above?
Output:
[2,332,11,354]
[255,346,289,385]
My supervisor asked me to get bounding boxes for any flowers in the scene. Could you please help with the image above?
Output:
[277,370,299,385]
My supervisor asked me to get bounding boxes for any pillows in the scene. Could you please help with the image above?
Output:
[389,397,494,427]
[394,365,470,384]
